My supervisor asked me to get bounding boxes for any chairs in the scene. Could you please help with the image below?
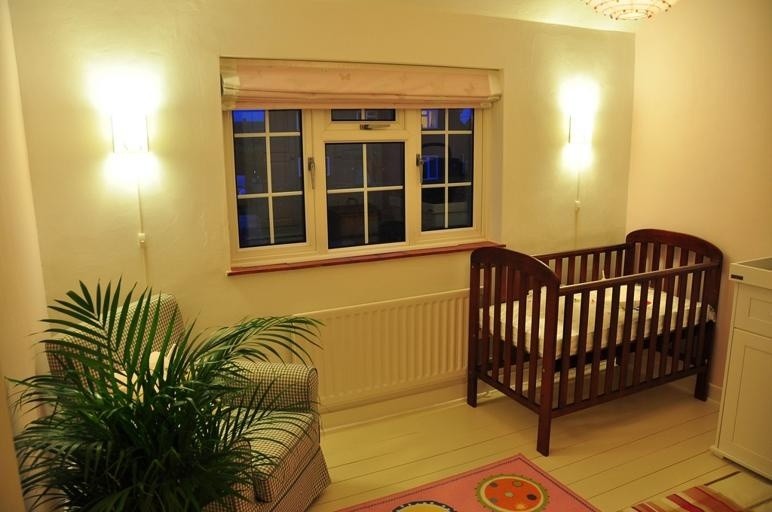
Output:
[46,292,332,511]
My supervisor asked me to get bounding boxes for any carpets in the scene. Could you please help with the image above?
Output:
[624,485,745,512]
[332,453,598,511]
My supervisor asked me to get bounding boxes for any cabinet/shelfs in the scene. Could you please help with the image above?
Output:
[710,283,772,483]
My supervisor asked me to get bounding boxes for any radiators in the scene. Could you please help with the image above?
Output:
[291,285,488,411]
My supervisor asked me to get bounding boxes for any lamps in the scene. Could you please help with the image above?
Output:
[585,2,673,24]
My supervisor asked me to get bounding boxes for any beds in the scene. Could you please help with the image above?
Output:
[466,228,724,455]
